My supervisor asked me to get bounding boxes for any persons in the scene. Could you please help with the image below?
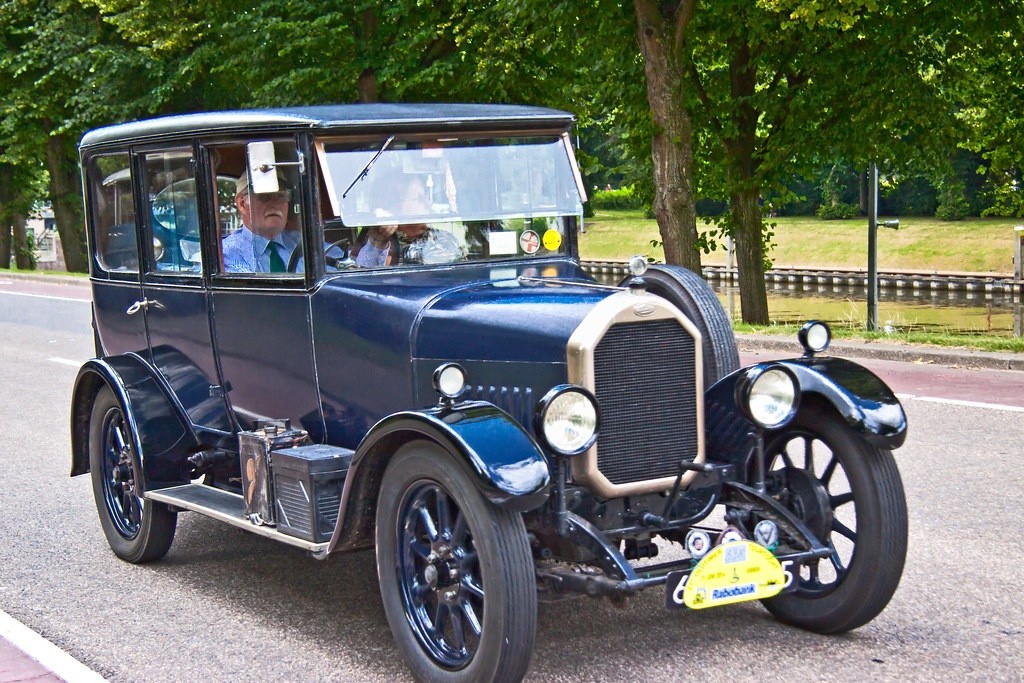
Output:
[352,170,469,276]
[191,164,399,279]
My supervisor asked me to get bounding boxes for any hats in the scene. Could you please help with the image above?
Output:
[236,166,287,194]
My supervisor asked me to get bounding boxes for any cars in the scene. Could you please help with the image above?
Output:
[67,101,914,682]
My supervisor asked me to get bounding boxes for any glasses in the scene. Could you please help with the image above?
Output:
[241,188,293,204]
